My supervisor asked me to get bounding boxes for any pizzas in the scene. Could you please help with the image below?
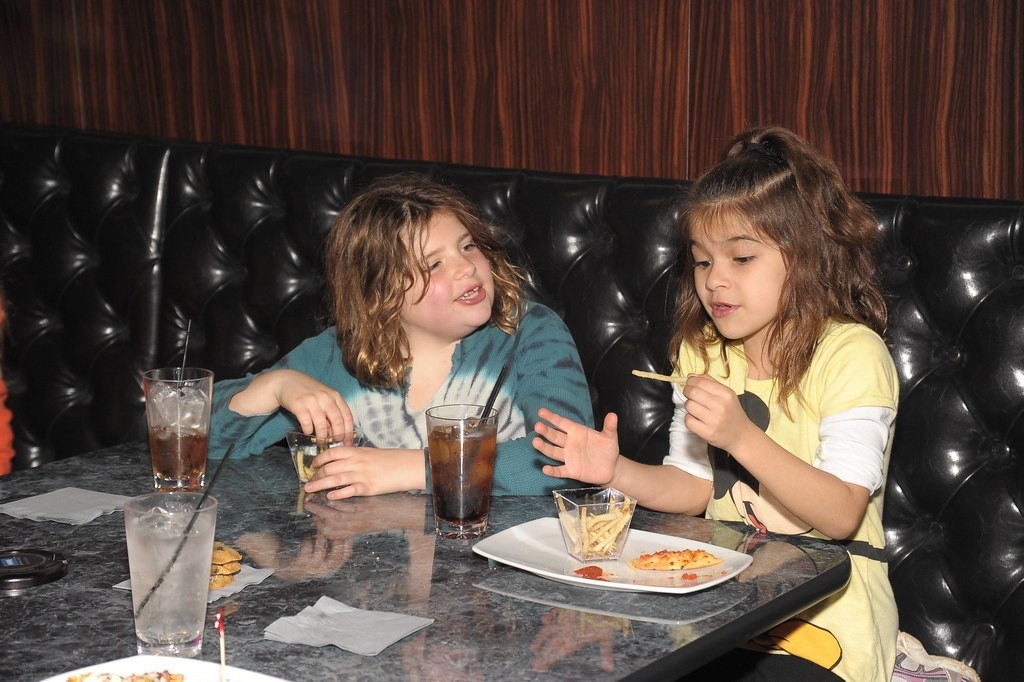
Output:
[64,668,185,682]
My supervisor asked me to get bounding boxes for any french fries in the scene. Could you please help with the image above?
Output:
[632,370,688,383]
[557,492,632,554]
[296,432,360,479]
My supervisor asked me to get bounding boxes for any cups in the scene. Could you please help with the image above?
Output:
[124,491,218,657]
[284,424,362,484]
[143,367,214,492]
[552,487,637,562]
[426,404,498,539]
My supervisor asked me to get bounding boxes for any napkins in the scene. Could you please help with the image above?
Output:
[263,596,435,656]
[0,487,151,526]
[111,564,278,605]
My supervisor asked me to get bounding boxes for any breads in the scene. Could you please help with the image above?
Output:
[209,541,243,589]
[633,548,724,571]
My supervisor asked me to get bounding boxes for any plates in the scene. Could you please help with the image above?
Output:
[473,517,753,594]
[39,655,288,682]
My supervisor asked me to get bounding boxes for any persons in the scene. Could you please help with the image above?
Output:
[209,457,848,682]
[532,128,899,682]
[207,175,594,500]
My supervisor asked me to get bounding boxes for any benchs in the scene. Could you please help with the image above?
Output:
[0,120,1024,682]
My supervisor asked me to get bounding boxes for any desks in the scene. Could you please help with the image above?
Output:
[0,437,852,682]
